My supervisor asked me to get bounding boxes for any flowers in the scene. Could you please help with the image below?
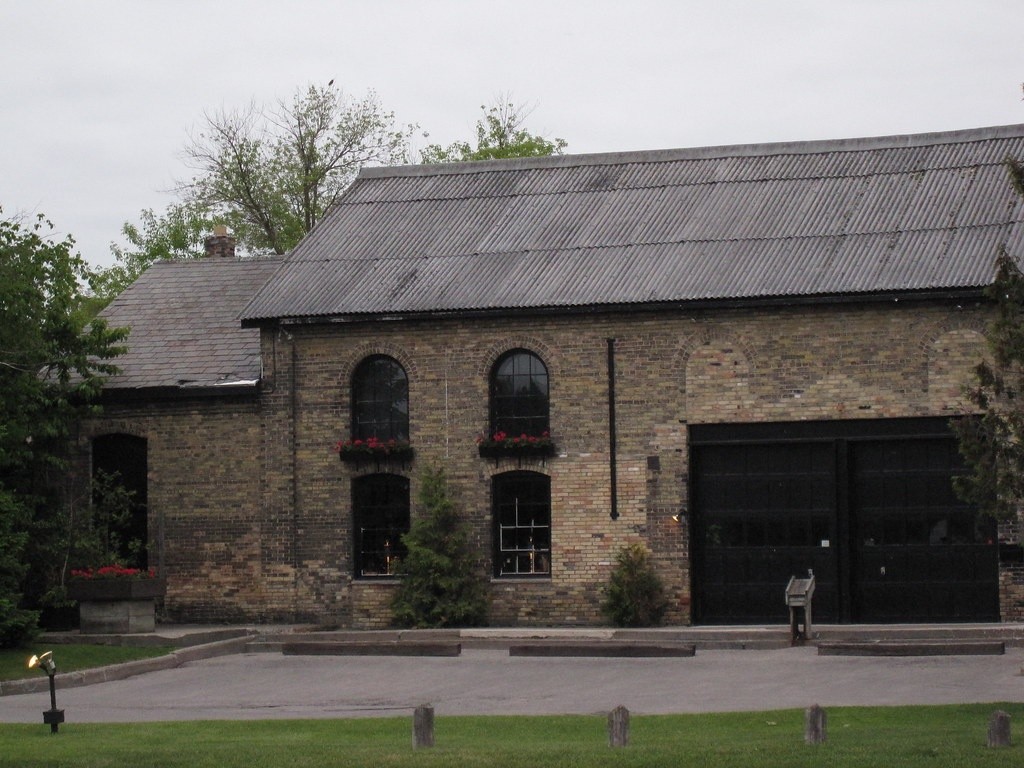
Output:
[339,433,416,465]
[71,564,167,581]
[477,430,559,461]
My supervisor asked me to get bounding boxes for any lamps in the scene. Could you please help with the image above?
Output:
[672,508,688,522]
[27,649,57,677]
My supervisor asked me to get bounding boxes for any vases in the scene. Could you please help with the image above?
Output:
[74,579,168,604]
